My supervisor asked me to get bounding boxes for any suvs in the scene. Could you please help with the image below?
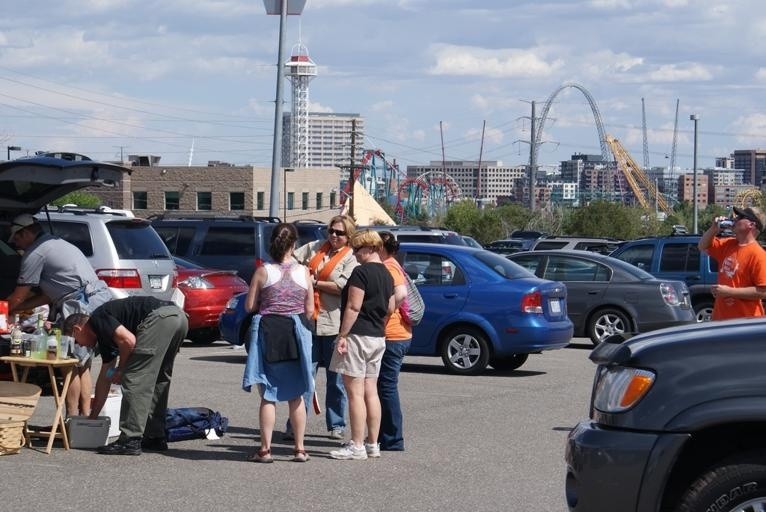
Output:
[566,318,763,506]
[0,154,131,308]
[31,206,186,342]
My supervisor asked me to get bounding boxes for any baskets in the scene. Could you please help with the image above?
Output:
[0,381,42,423]
[0,422,26,455]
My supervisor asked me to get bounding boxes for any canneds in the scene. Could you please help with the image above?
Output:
[718,220,733,230]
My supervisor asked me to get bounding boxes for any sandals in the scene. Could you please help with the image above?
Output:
[293,450,310,461]
[247,448,273,463]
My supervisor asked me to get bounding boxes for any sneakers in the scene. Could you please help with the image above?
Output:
[142,437,168,451]
[329,440,368,461]
[283,431,294,440]
[364,443,381,457]
[331,427,344,439]
[96,440,141,455]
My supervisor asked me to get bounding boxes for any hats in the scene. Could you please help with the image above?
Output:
[733,207,762,232]
[7,213,41,243]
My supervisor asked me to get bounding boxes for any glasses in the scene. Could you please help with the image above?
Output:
[354,246,362,252]
[328,228,345,236]
[72,329,78,344]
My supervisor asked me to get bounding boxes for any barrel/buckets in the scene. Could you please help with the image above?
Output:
[86,390,123,439]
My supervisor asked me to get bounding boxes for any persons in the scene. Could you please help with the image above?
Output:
[284,215,362,441]
[693,205,766,325]
[245,223,315,461]
[4,214,120,428]
[374,232,428,453]
[329,229,395,459]
[58,296,191,457]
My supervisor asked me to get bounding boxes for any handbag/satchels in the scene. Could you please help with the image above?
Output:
[166,407,228,442]
[384,261,426,326]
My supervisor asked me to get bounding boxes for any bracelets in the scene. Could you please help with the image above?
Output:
[314,280,318,288]
[338,333,347,338]
[708,226,717,235]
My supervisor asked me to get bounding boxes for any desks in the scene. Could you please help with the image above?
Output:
[0,353,81,454]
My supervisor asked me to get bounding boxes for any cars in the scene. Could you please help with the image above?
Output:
[222,242,575,375]
[169,254,245,342]
[506,251,696,344]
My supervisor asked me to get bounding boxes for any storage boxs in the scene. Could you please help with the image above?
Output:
[89,395,124,439]
[66,416,111,449]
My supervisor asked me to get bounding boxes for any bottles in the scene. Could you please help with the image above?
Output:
[8,314,24,356]
[30,315,46,358]
[51,324,61,359]
[46,330,56,359]
